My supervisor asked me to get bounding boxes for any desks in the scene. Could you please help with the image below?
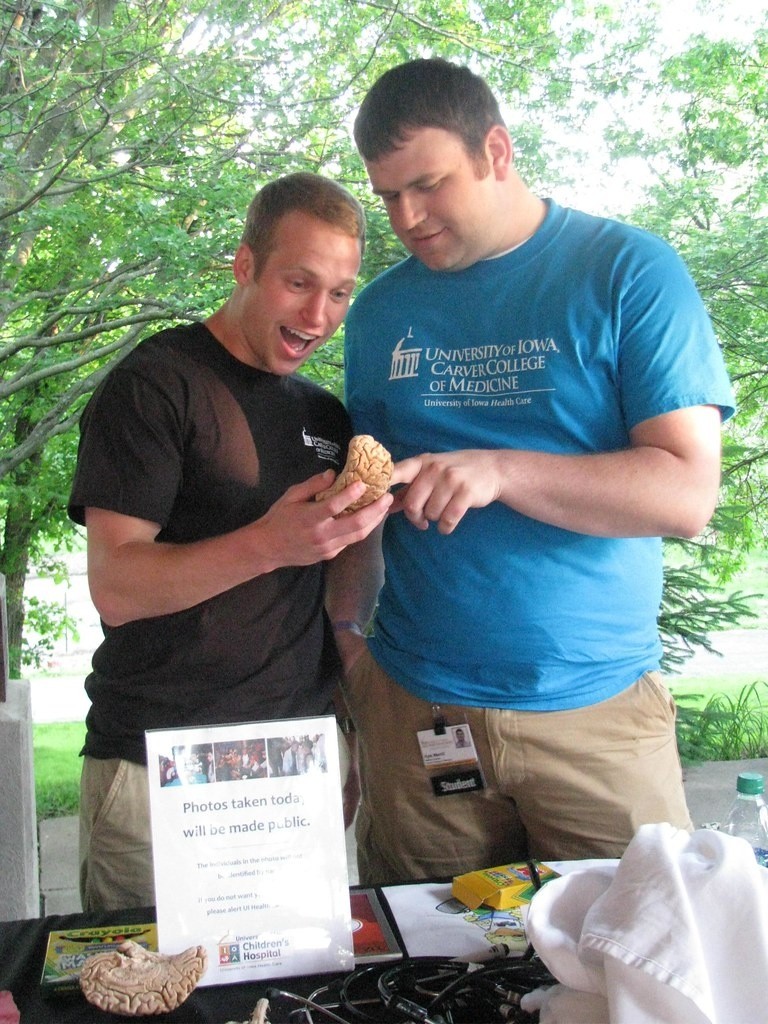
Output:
[0,855,623,1024]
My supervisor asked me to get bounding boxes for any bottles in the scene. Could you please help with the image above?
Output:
[719,771,768,868]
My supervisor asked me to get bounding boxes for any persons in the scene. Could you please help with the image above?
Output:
[329,57,737,890]
[69,171,391,919]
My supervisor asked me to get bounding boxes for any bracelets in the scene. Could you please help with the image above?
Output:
[331,618,363,637]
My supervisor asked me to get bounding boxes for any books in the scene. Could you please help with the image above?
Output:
[349,888,403,965]
